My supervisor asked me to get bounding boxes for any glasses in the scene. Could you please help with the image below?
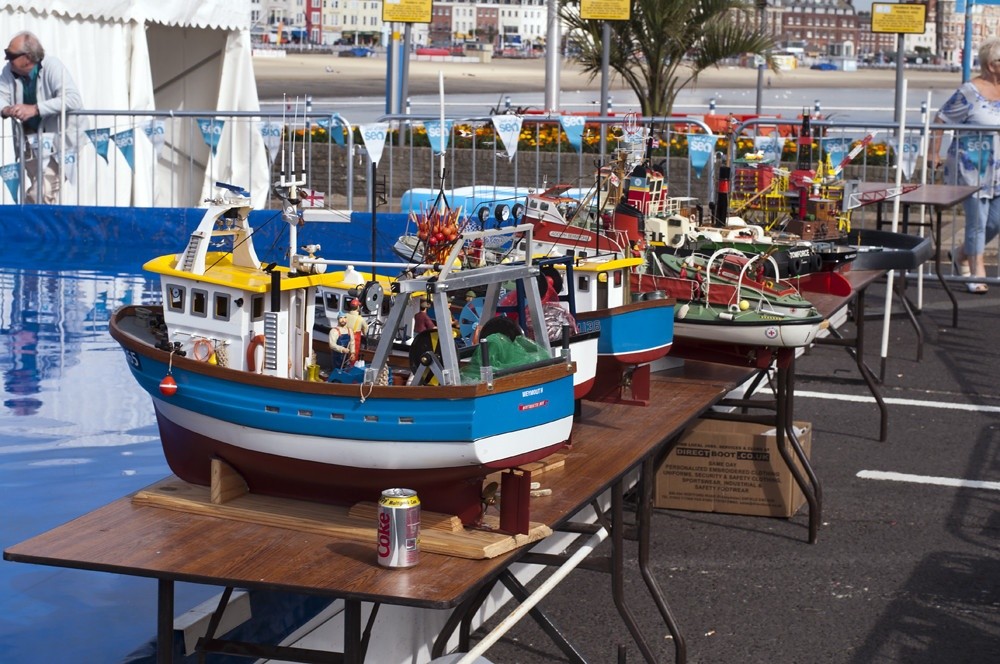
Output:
[4,49,26,61]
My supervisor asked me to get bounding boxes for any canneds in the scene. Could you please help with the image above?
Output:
[376,487,421,570]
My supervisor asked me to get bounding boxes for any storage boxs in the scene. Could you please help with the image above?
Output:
[655,418,818,523]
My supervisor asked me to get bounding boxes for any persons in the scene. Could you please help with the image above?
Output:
[329,312,354,369]
[345,299,369,365]
[447,290,477,339]
[414,302,435,338]
[566,207,574,218]
[928,39,1000,294]
[0,31,89,205]
[601,211,612,231]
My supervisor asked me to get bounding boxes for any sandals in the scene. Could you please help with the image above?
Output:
[965,282,988,295]
[948,251,971,277]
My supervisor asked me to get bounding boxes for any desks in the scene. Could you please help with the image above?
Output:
[802,165,986,347]
[4,351,763,664]
[642,248,900,555]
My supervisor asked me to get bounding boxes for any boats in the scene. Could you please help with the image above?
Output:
[108,160,856,533]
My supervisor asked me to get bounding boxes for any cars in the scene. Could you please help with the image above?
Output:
[809,63,838,70]
[339,46,375,57]
